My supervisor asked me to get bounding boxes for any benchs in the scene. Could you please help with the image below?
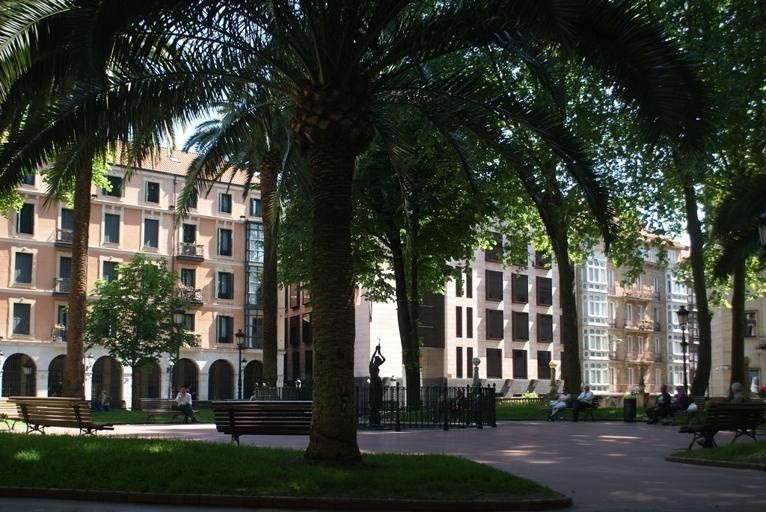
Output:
[544,394,602,421]
[670,396,766,451]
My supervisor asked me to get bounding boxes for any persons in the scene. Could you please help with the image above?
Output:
[175,385,199,423]
[572,385,594,421]
[731,382,751,399]
[548,384,573,422]
[95,389,113,411]
[661,386,689,425]
[645,383,672,424]
[368,345,386,424]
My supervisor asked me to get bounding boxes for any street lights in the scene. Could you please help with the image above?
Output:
[168,355,177,400]
[171,298,187,398]
[470,355,482,387]
[234,327,246,401]
[547,358,558,385]
[240,356,247,400]
[294,378,303,401]
[675,304,691,397]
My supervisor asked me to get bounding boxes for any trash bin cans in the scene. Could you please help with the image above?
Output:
[624,397,637,422]
